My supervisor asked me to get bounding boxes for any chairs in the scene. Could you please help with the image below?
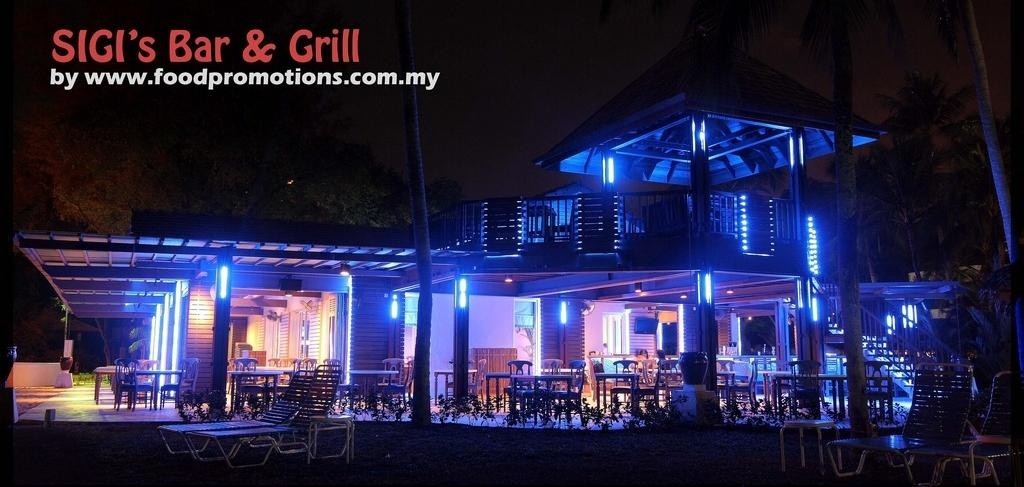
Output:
[186,362,346,470]
[823,351,976,479]
[91,357,900,422]
[153,368,315,463]
[897,364,1023,487]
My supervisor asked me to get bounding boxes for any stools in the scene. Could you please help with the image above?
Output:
[302,411,358,466]
[778,415,844,476]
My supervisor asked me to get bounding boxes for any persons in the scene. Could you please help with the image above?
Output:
[637,349,667,373]
